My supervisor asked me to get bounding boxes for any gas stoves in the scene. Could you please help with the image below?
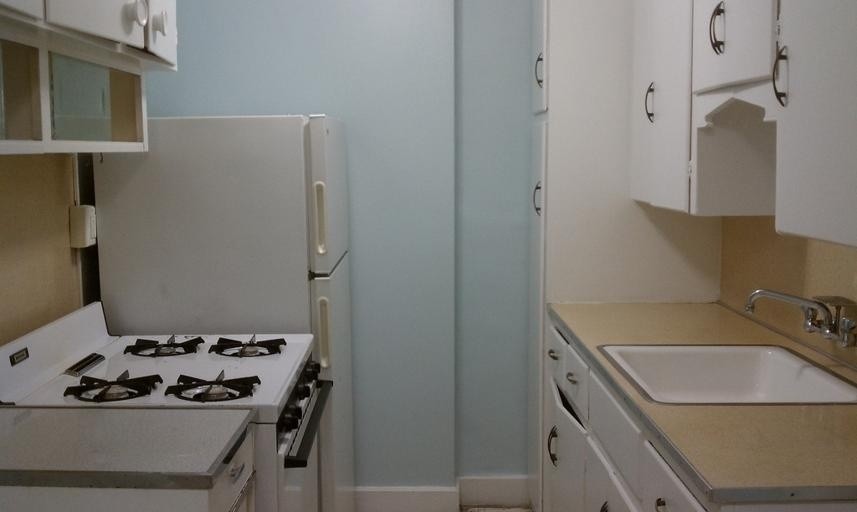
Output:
[15,333,322,432]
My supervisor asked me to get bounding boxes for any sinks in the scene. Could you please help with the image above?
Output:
[600,343,857,405]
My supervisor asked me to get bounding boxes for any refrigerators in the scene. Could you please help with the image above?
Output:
[92,114,355,512]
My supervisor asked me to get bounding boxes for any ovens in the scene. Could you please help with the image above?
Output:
[277,378,336,512]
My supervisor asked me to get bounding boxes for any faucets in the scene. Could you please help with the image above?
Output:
[744,289,833,330]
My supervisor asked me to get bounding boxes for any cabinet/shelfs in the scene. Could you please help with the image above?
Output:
[588,371,707,512]
[628,0,775,217]
[524,1,721,512]
[46,0,178,74]
[538,324,588,512]
[775,1,857,247]
